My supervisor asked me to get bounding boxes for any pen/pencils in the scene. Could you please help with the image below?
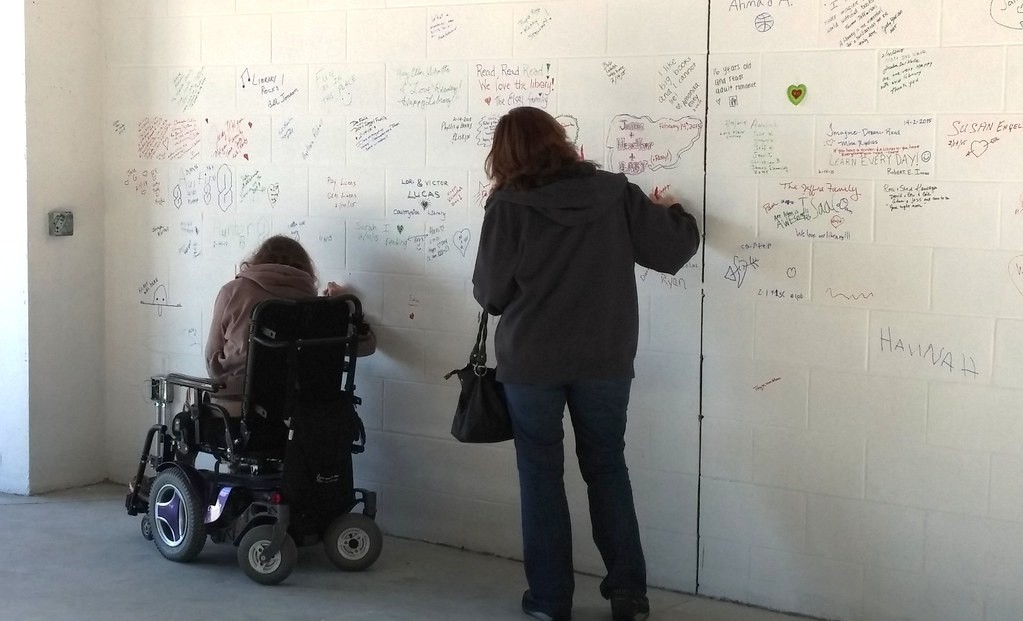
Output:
[325,291,329,297]
[654,187,658,197]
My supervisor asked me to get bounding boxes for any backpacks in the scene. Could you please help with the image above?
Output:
[282,333,368,540]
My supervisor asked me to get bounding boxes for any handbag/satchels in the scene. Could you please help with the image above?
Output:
[442,309,515,444]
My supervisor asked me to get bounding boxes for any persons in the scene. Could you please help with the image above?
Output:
[470,106,700,621]
[204,236,377,418]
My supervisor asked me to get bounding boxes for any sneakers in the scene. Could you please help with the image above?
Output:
[521,589,571,621]
[610,590,649,621]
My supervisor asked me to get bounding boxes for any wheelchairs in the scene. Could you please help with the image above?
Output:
[125,293,385,585]
